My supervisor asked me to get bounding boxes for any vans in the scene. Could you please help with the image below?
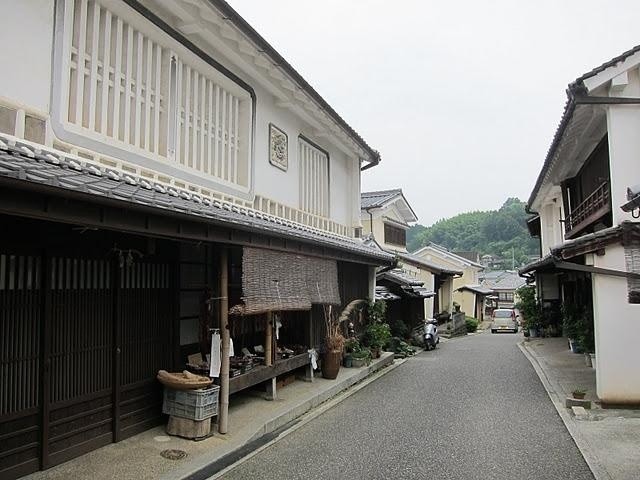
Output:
[490,308,519,333]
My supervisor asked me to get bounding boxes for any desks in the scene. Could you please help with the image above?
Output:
[203,352,314,398]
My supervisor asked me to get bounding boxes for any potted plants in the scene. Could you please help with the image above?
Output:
[512,285,542,338]
[571,384,590,399]
[344,299,391,367]
[563,315,596,369]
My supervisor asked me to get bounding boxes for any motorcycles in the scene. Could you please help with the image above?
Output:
[422,316,439,350]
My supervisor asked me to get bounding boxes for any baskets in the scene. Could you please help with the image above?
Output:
[156,368,215,389]
[161,382,220,422]
